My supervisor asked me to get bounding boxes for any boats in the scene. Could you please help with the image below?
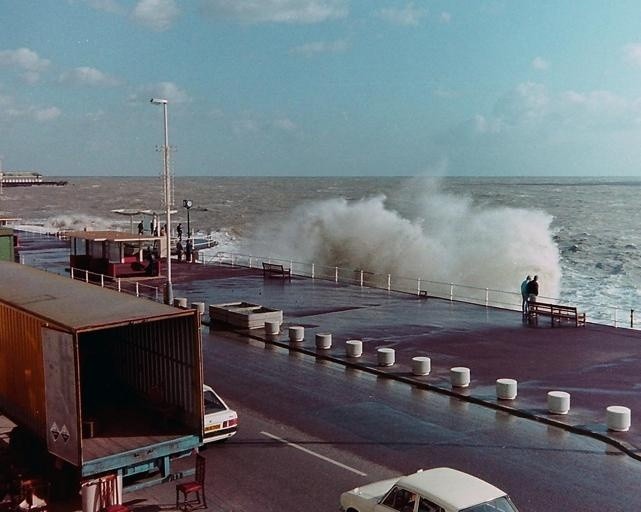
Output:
[0,169,70,188]
[139,208,179,217]
[110,206,142,216]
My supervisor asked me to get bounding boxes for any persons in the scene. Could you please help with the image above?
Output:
[177,223,183,241]
[527,275,539,317]
[176,239,183,262]
[521,276,531,315]
[164,224,167,232]
[137,220,144,235]
[154,227,158,236]
[185,239,193,263]
[150,223,153,235]
[160,227,165,236]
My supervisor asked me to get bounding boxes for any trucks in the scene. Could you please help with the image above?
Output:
[0,258,208,505]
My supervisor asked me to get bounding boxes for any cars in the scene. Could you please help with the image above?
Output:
[334,465,523,512]
[200,382,241,451]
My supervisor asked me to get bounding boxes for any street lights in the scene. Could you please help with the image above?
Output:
[145,94,174,308]
[181,199,192,238]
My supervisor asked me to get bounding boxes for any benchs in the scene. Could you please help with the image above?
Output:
[526,299,586,328]
[263,260,291,280]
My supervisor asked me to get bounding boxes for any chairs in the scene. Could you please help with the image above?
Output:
[16,477,56,511]
[174,451,207,512]
[97,472,130,511]
